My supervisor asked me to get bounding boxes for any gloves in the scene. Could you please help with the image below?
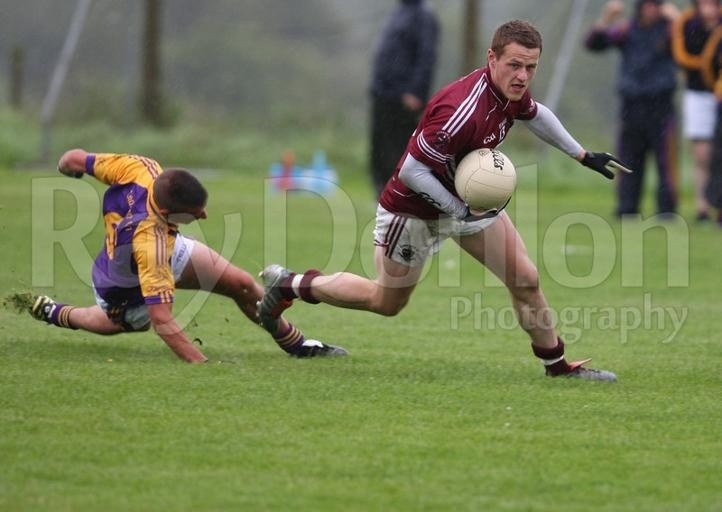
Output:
[55,163,84,180]
[579,149,634,181]
[460,194,514,224]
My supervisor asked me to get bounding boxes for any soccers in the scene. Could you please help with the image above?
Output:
[454,148,517,211]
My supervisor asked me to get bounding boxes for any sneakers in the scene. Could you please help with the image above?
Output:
[292,339,348,358]
[543,357,619,384]
[27,294,57,325]
[255,264,294,334]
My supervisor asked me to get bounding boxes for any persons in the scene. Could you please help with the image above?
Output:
[582,1,685,223]
[368,0,442,195]
[27,147,350,365]
[671,2,722,223]
[256,20,632,383]
